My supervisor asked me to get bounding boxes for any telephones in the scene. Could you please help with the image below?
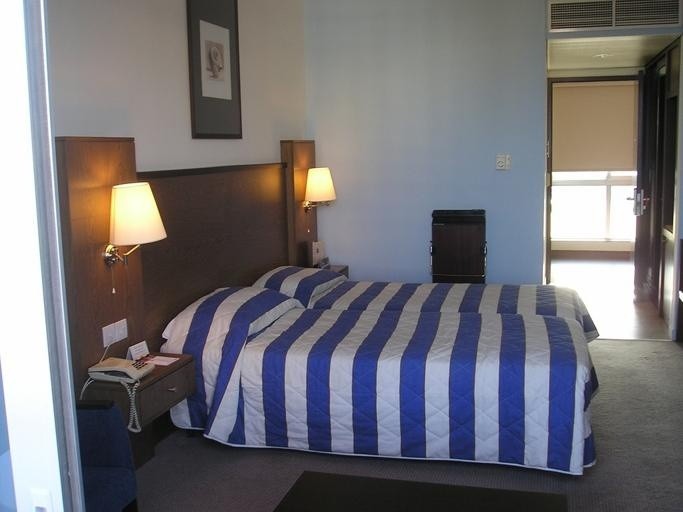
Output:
[87,357,155,384]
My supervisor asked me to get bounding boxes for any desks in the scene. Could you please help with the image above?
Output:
[267,468,573,512]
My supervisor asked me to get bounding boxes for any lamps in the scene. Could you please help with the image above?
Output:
[303,164,335,214]
[99,179,169,265]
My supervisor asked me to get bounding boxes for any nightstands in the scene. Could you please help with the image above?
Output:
[309,256,346,275]
[92,350,197,471]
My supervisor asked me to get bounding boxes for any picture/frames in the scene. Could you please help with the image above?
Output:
[185,1,243,140]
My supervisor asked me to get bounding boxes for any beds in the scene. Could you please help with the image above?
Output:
[132,138,604,477]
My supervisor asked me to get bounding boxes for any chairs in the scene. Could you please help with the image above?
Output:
[77,397,138,512]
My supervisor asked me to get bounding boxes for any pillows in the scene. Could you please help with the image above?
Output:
[257,255,347,301]
[159,284,292,340]
